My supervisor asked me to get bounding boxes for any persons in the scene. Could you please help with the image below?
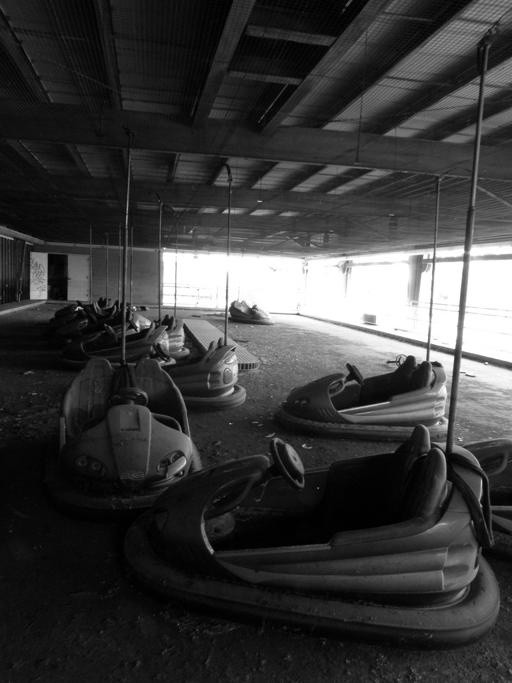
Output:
[104,302,129,327]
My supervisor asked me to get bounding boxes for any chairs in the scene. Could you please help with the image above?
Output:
[360,355,416,396]
[160,313,169,326]
[334,448,447,544]
[134,359,187,431]
[218,337,226,346]
[329,423,430,522]
[167,315,176,332]
[58,356,116,439]
[411,361,433,391]
[231,300,251,317]
[198,341,217,363]
